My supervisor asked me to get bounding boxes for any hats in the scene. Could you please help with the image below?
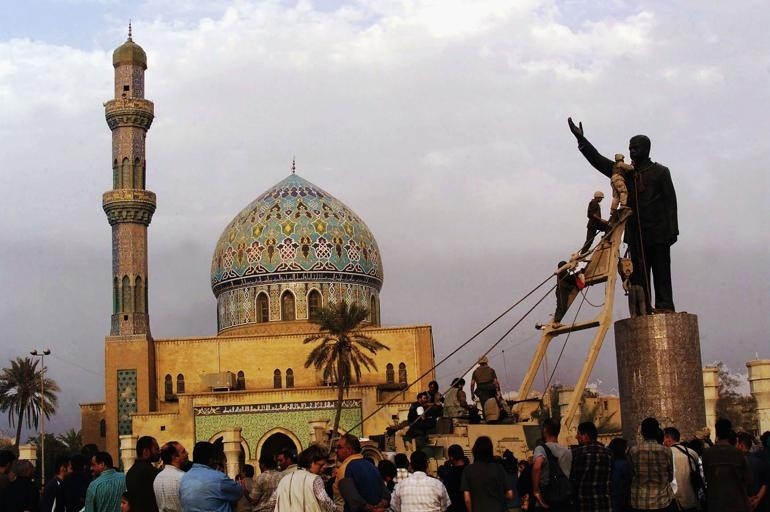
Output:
[417,407,424,416]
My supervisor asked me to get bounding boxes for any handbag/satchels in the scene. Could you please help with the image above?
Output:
[544,455,570,506]
[691,471,706,500]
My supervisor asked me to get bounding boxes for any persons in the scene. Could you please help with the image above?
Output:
[551,261,584,329]
[579,191,612,255]
[234,418,770,511]
[567,117,679,318]
[627,255,647,317]
[2,435,243,510]
[404,357,501,447]
[610,153,635,213]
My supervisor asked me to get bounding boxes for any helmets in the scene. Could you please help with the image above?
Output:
[594,191,604,198]
[615,154,624,160]
[479,357,488,363]
